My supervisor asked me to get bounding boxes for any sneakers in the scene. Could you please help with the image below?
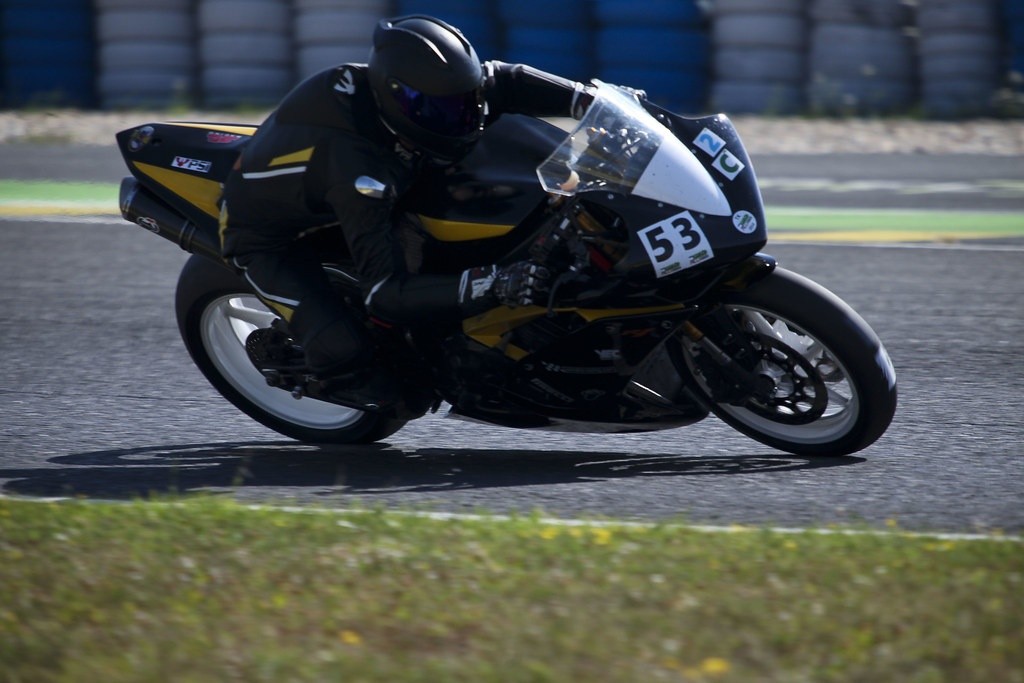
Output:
[319,360,404,412]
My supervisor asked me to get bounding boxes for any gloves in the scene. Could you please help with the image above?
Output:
[571,81,647,121]
[456,260,549,309]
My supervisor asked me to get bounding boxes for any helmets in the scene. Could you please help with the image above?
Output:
[367,14,489,166]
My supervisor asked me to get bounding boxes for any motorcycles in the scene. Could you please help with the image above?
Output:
[115,80,897,458]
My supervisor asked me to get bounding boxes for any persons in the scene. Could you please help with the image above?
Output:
[214,13,647,406]
